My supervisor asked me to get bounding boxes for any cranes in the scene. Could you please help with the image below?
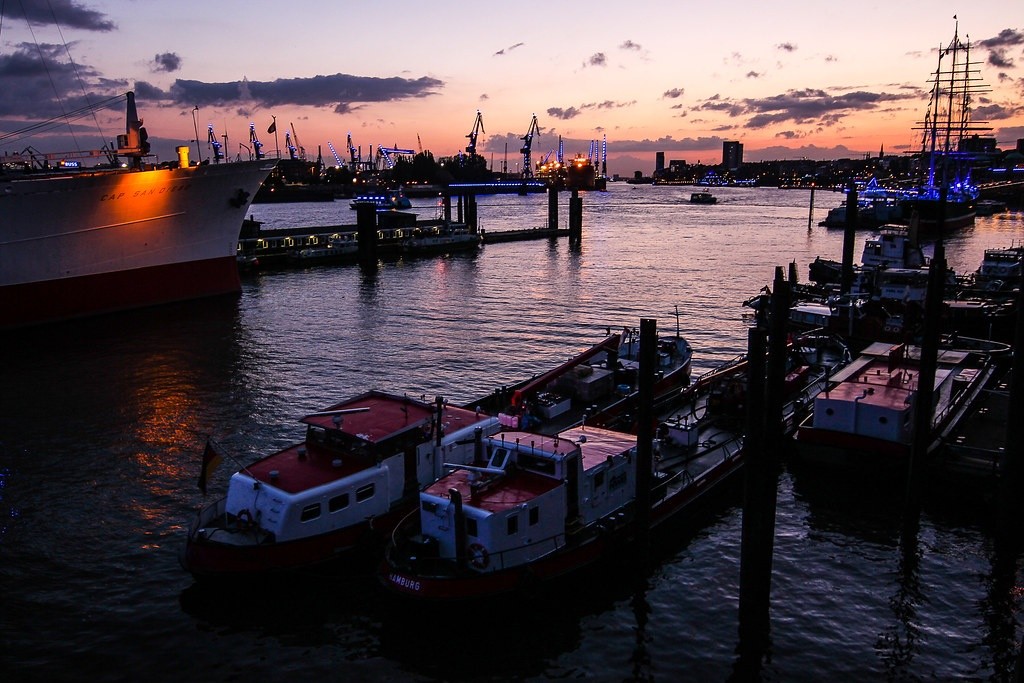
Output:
[465,112,485,163]
[519,115,541,183]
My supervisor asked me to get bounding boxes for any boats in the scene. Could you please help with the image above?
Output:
[181,308,693,592]
[349,184,412,210]
[386,324,854,614]
[690,193,717,205]
[0,0,283,287]
[740,180,1023,494]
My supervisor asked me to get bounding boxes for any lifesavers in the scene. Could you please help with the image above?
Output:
[468,543,490,570]
[237,510,252,531]
